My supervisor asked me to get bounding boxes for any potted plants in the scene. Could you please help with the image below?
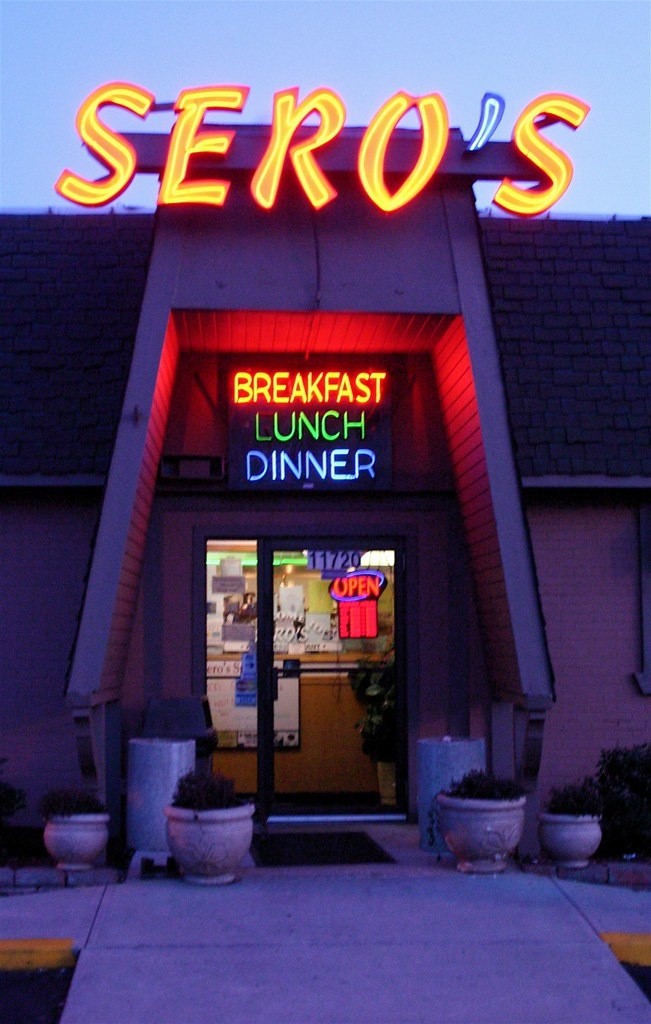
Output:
[429,768,526,873]
[165,765,256,884]
[38,785,111,872]
[348,643,398,806]
[536,776,604,869]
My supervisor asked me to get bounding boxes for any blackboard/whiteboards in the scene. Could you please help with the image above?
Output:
[204,658,301,751]
